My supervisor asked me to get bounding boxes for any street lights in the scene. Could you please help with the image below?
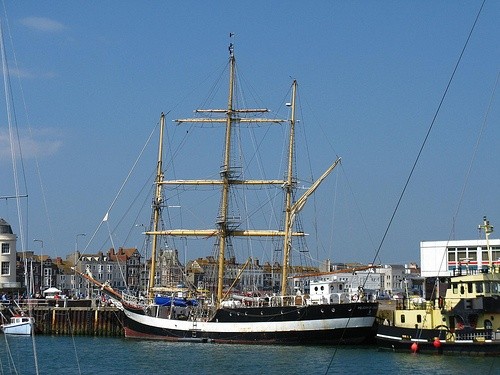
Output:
[32,238,43,298]
[75,232,87,295]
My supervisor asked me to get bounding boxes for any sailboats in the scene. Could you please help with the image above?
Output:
[71,32,382,349]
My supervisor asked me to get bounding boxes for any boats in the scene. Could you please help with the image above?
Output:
[0,316,35,336]
[376,217,500,351]
